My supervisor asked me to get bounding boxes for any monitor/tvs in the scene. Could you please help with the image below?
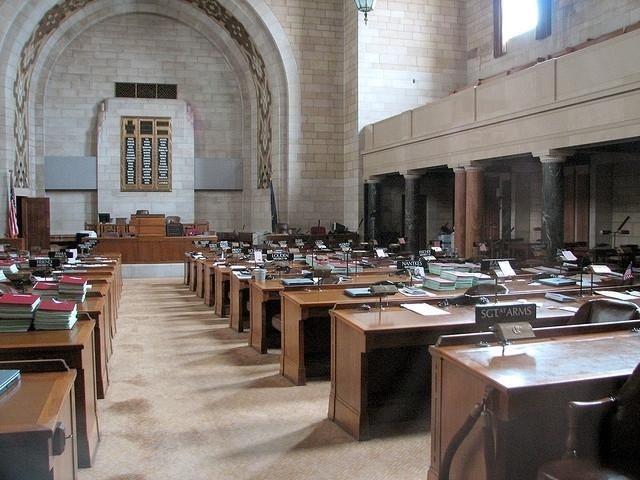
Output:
[99,214,110,224]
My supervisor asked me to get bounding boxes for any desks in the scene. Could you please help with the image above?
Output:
[0,249,123,480]
[82,236,218,264]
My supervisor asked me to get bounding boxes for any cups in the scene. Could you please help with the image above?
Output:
[253,268,267,283]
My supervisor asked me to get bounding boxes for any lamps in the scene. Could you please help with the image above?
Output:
[355,0,376,25]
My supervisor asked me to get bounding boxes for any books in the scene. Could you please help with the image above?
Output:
[189,228,624,291]
[1,242,112,332]
